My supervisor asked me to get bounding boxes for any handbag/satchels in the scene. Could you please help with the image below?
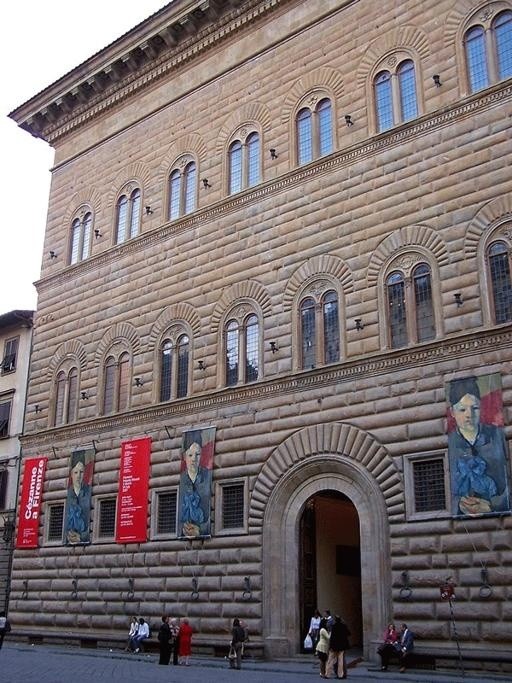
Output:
[304,637,313,648]
[229,653,237,658]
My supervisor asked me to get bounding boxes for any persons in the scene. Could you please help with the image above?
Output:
[234,621,248,658]
[62,452,92,543]
[303,609,352,680]
[169,618,180,665]
[158,615,174,665]
[177,619,192,666]
[132,618,150,654]
[124,617,139,651]
[0,611,7,650]
[447,377,509,518]
[393,624,414,669]
[380,623,398,670]
[177,430,212,537]
[228,618,245,670]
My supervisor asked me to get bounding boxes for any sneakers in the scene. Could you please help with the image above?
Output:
[381,665,387,670]
[400,666,406,672]
[403,650,409,657]
